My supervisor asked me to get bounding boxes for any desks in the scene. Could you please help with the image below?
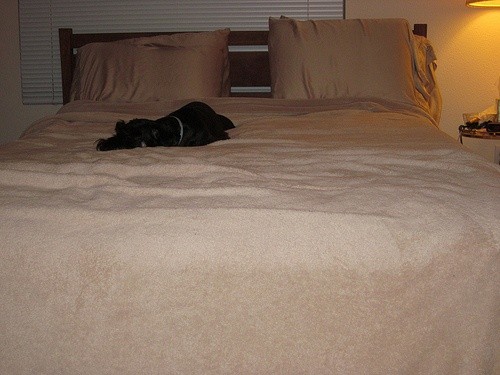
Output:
[457,125,500,144]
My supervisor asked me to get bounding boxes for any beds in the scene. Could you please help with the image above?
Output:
[0,23,500,375]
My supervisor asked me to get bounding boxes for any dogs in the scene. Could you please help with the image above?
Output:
[90,101,239,154]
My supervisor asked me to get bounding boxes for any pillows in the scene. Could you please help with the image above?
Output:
[268,15,417,104]
[71,27,232,99]
